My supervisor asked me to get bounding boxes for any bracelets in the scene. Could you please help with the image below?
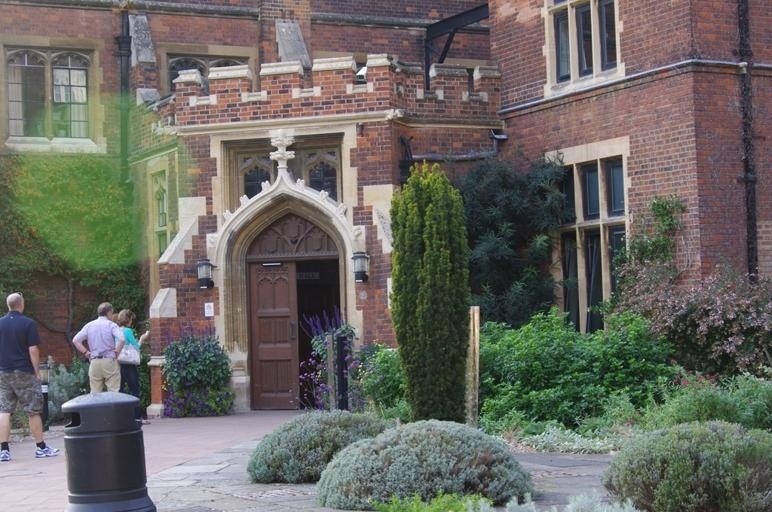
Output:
[83,349,88,356]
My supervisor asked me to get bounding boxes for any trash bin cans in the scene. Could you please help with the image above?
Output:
[61,391,157,512]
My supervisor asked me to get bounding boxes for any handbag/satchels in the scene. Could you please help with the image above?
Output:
[117,344,141,366]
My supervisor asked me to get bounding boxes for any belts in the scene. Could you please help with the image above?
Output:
[91,355,103,359]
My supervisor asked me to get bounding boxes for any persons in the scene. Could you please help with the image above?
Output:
[0,290,60,462]
[69,301,126,395]
[115,308,151,425]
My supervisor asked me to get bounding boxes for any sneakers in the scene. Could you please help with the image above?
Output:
[35,441,60,458]
[141,419,150,424]
[0,449,12,461]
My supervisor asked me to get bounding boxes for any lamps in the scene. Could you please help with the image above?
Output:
[196,259,216,291]
[351,250,369,286]
